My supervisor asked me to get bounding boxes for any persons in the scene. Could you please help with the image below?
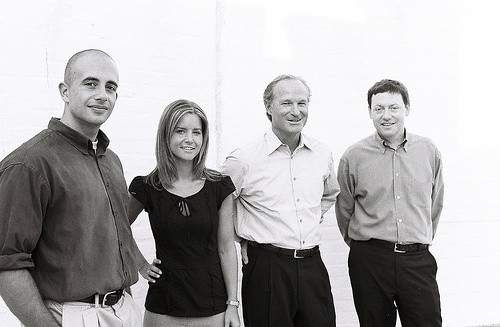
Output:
[220,74,341,327]
[127,99,241,327]
[0,50,143,327]
[335,80,444,327]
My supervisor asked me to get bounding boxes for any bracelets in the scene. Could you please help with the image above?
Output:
[226,301,240,308]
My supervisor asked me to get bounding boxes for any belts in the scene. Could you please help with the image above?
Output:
[248,243,320,258]
[370,239,428,253]
[77,289,124,308]
